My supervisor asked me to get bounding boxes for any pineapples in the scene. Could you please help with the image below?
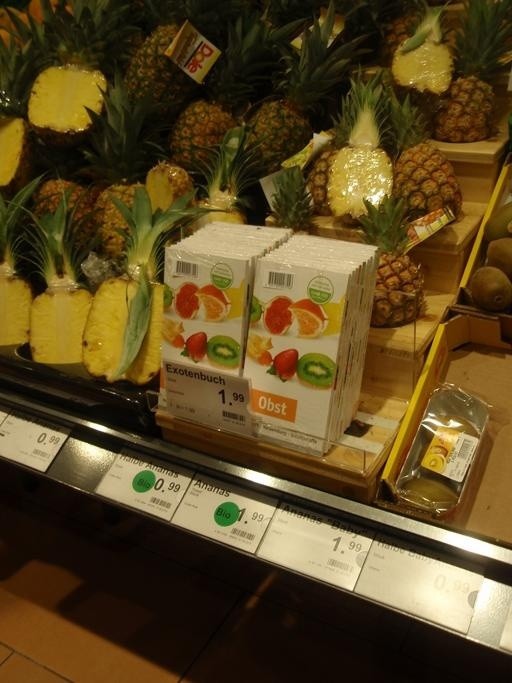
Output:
[1,2,497,386]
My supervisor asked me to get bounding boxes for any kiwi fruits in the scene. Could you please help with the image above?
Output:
[400,412,480,514]
[470,199,511,313]
